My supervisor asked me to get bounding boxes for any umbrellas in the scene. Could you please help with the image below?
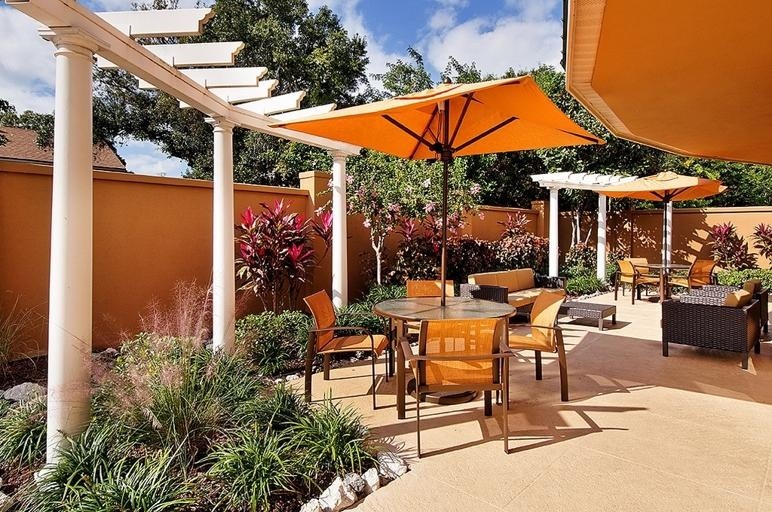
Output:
[266,74,605,309]
[593,171,728,301]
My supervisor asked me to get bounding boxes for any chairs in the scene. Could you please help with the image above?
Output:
[498,288,569,410]
[303,289,389,410]
[460,283,508,305]
[615,260,646,304]
[663,294,760,370]
[396,318,515,458]
[697,278,768,335]
[624,257,656,295]
[670,259,719,296]
[406,280,454,297]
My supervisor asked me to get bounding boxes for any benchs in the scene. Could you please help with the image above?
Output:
[467,268,566,321]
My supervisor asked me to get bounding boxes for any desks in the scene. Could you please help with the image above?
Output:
[374,296,518,400]
[632,263,690,306]
[559,302,616,330]
